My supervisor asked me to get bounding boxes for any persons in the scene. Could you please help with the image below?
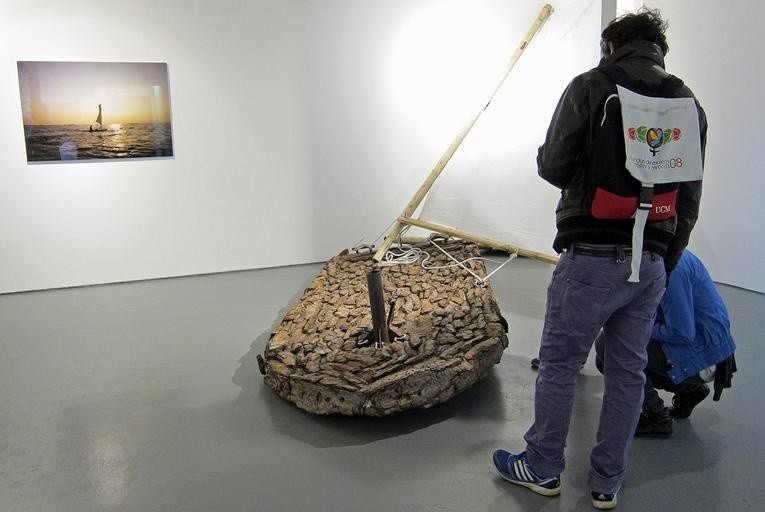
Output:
[491,6,711,509]
[592,250,736,437]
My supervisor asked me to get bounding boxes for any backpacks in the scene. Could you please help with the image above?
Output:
[586,64,704,222]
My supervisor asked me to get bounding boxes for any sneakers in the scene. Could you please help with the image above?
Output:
[589,485,622,509]
[633,410,675,440]
[669,383,711,419]
[493,449,562,497]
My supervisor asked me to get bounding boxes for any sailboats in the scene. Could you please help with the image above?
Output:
[84,104,107,132]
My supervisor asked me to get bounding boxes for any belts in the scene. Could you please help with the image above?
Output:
[568,244,632,258]
